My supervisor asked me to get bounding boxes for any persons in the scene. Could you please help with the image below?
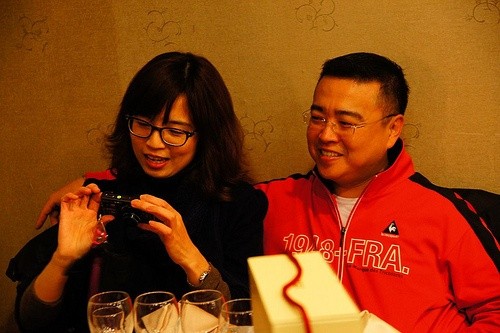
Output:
[17,52,269,333]
[36,53,500,333]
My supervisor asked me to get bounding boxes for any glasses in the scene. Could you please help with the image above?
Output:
[301,109,396,137]
[123,115,201,147]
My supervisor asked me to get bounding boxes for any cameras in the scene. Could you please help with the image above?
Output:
[101,191,147,224]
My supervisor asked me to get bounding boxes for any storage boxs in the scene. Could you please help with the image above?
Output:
[247,251,364,333]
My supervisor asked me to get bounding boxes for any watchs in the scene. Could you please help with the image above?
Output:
[189,264,211,287]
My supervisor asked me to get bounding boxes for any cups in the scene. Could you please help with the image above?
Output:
[87,290,134,333]
[132,291,180,333]
[220,298,256,333]
[180,289,226,333]
[92,307,124,333]
